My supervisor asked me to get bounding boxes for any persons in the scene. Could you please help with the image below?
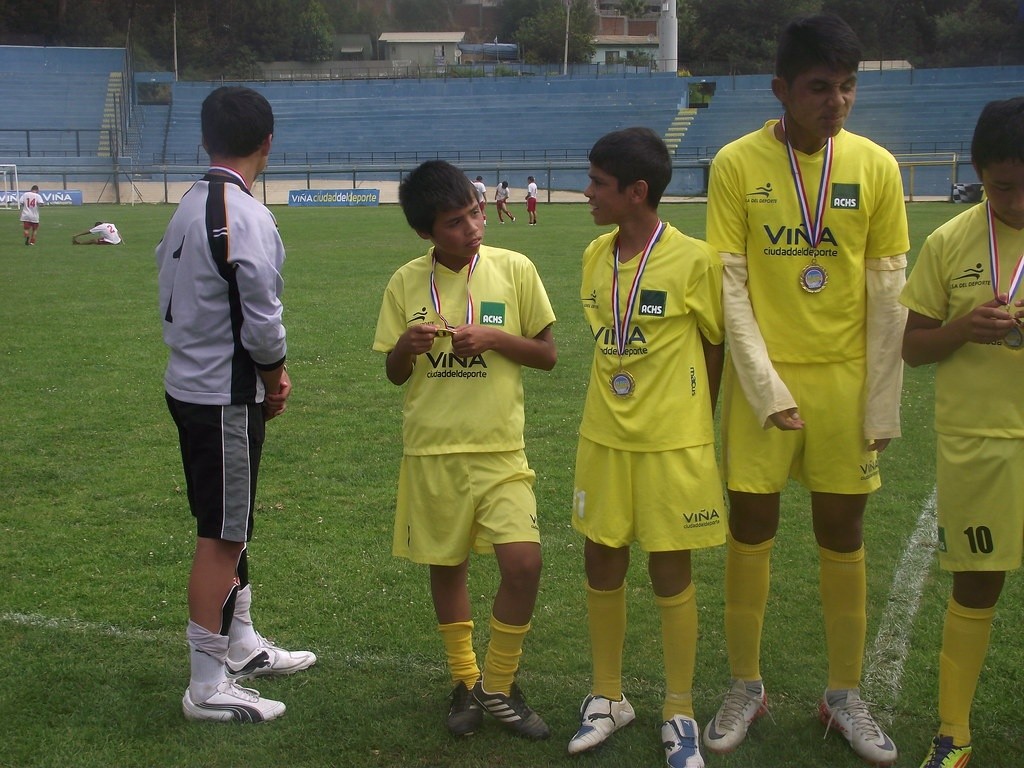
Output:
[471,176,489,226]
[494,181,515,225]
[372,161,559,739]
[703,13,911,767]
[20,185,45,246]
[157,84,317,723]
[524,176,538,226]
[897,95,1024,768]
[71,221,125,246]
[569,126,727,768]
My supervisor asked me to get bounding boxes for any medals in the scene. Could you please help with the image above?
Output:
[434,329,458,337]
[1002,326,1023,350]
[800,263,828,293]
[609,369,636,399]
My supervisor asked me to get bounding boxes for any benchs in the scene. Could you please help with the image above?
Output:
[0,45,1024,168]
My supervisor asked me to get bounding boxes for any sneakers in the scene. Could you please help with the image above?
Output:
[448,672,551,741]
[568,692,636,754]
[660,712,704,768]
[224,630,317,681]
[819,687,898,766]
[920,734,972,768]
[702,679,766,754]
[182,678,286,723]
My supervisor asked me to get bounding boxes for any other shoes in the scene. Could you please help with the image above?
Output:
[25,237,35,246]
